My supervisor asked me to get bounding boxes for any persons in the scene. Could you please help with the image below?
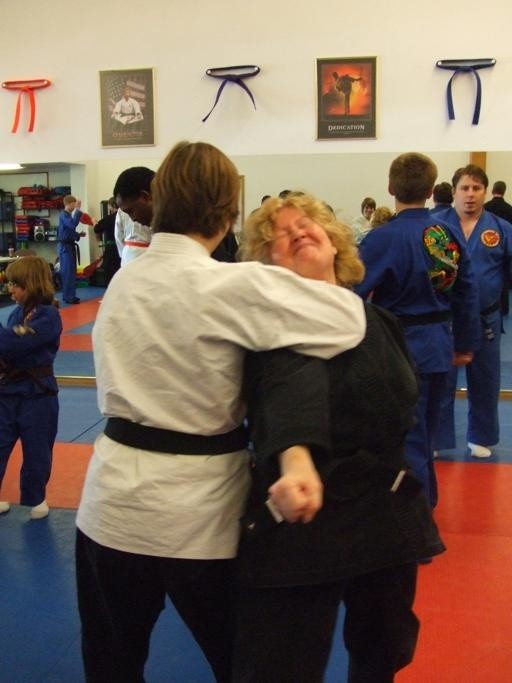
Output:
[111,89,144,134]
[57,167,238,303]
[76,139,367,682]
[235,192,446,682]
[347,154,511,508]
[2,257,62,521]
[331,70,363,113]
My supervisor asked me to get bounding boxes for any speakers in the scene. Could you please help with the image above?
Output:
[32,224,45,242]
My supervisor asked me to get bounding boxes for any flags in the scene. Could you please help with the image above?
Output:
[123,77,146,109]
[105,76,126,111]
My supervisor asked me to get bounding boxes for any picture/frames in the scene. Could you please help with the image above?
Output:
[99,66,156,148]
[314,55,378,138]
